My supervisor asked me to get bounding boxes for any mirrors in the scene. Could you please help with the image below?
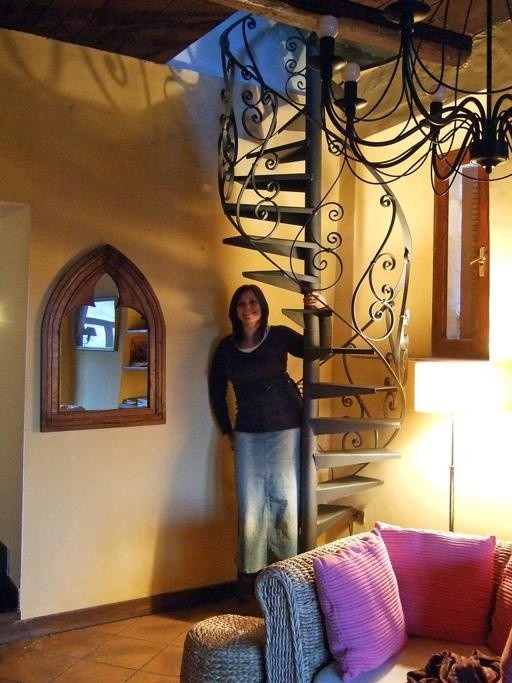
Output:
[40,243,166,433]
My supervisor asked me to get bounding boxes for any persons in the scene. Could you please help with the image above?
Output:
[206,285,331,576]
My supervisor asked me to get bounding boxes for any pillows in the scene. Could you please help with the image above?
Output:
[313,528,409,683]
[374,520,497,646]
[488,555,511,655]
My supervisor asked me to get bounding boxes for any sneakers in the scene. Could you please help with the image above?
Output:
[237,572,257,600]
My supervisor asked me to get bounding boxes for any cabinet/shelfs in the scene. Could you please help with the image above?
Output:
[118,307,149,408]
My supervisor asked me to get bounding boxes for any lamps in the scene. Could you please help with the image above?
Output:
[413,360,500,531]
[315,0,511,196]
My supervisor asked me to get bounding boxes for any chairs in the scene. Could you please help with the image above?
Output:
[253,531,511,683]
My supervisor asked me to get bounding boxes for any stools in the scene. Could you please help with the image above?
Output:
[180,612,266,683]
